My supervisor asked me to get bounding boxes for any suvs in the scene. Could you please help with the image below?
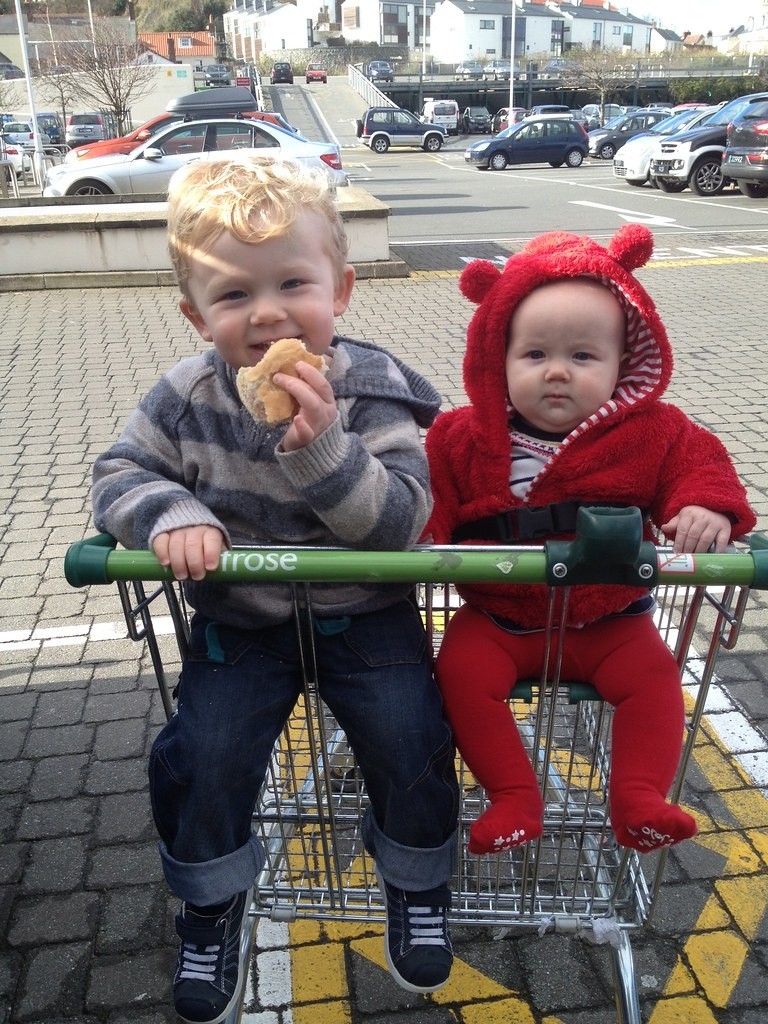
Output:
[587,111,671,160]
[649,92,768,196]
[719,92,768,198]
[65,111,107,149]
[1,119,41,148]
[65,111,302,164]
[0,132,32,182]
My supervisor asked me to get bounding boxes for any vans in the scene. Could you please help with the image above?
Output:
[269,61,293,84]
[418,100,459,136]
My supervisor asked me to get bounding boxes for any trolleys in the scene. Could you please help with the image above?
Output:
[62,533,767,1024]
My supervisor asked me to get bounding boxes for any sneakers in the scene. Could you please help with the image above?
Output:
[375,862,453,993]
[173,887,253,1024]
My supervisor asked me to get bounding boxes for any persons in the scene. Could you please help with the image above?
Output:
[91,151,455,1023]
[419,223,759,849]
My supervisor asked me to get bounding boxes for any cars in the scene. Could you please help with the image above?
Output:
[464,116,590,171]
[483,60,521,81]
[30,111,64,145]
[454,61,485,81]
[544,58,583,78]
[367,60,394,83]
[462,106,493,134]
[42,116,350,198]
[0,63,25,80]
[612,107,721,190]
[355,107,449,154]
[305,63,327,84]
[491,102,710,133]
[204,64,231,87]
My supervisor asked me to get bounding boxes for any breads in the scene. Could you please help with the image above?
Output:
[234,335,330,427]
